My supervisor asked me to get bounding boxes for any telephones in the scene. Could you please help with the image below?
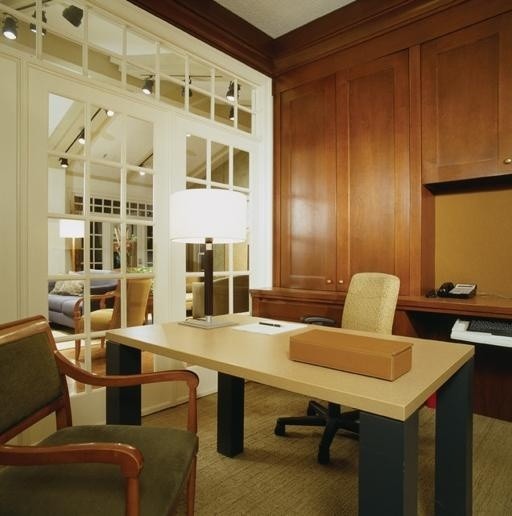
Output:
[437,282,477,297]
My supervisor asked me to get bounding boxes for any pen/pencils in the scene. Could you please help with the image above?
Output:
[259,322,280,327]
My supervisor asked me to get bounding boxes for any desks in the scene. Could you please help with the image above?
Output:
[106,316,475,516]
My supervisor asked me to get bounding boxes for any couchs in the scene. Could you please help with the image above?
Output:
[186,274,249,319]
[48,269,118,329]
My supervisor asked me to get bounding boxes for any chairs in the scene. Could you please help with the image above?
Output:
[274,273,401,465]
[72,278,152,361]
[0,314,199,516]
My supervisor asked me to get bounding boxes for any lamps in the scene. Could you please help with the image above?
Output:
[139,165,146,176]
[169,188,247,330]
[3,0,83,40]
[60,109,115,168]
[229,106,235,120]
[58,219,85,270]
[142,74,242,102]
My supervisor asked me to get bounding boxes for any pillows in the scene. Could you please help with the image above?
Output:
[58,280,84,296]
[49,281,65,295]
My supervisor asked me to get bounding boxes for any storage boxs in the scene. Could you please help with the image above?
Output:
[289,329,413,382]
